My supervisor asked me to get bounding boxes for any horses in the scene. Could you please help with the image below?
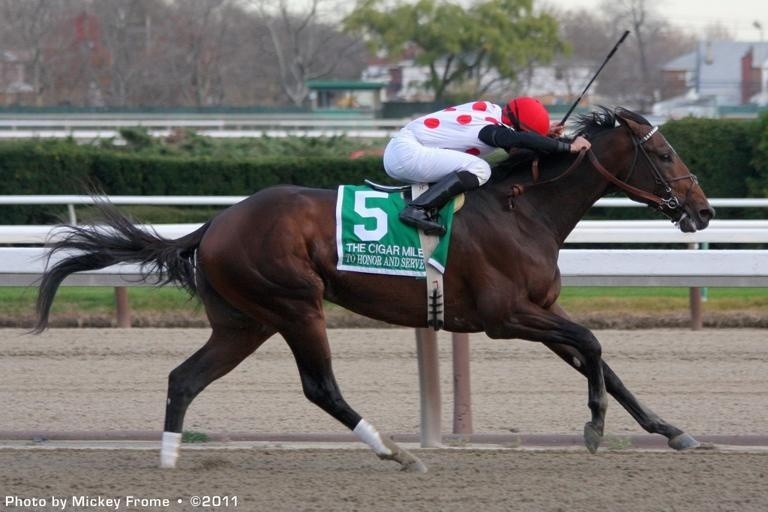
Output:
[18,105,716,474]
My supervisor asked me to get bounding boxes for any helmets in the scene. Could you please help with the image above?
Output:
[499,94,551,136]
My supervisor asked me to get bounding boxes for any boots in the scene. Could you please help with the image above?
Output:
[398,171,463,234]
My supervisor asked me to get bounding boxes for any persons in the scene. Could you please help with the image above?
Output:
[382,96,591,236]
